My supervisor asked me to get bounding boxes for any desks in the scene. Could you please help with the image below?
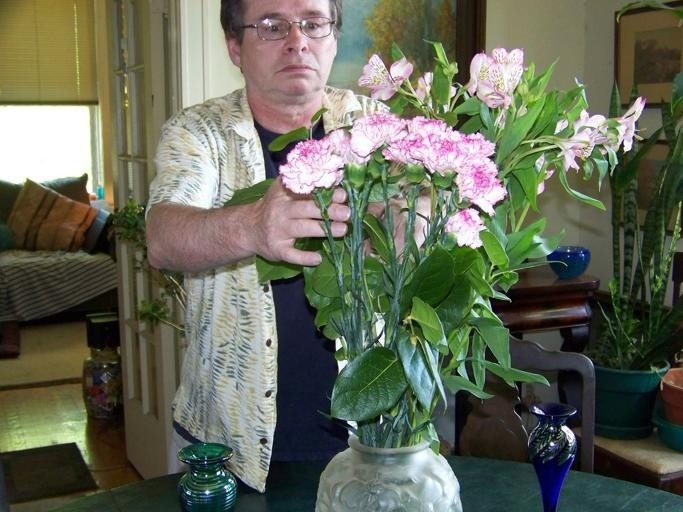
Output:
[52,449,681,511]
[453,265,599,471]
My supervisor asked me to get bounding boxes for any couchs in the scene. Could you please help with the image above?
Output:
[0,173,120,323]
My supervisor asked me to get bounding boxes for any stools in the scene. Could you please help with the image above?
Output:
[592,437,682,496]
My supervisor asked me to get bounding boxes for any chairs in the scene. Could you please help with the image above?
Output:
[440,333,596,474]
[568,248,682,496]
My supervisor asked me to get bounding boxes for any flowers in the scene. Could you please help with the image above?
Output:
[222,35,649,447]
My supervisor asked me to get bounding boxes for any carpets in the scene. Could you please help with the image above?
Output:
[0,442,98,505]
[0,321,119,390]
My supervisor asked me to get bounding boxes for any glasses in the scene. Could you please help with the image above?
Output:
[239,16,336,41]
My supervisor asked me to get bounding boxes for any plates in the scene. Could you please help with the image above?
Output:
[594,423,655,440]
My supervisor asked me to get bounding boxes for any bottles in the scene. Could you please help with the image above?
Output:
[512,403,580,511]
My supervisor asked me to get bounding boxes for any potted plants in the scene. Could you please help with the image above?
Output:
[581,79,681,438]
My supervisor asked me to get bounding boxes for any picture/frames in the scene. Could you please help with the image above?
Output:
[609,136,680,239]
[612,2,680,107]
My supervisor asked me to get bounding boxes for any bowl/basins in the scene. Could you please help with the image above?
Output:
[546,244,591,277]
[651,407,683,451]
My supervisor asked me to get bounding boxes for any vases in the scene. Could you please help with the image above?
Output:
[656,364,681,443]
[525,403,581,512]
[78,320,123,422]
[313,436,464,511]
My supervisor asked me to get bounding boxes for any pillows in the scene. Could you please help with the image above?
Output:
[0,173,91,222]
[5,178,97,253]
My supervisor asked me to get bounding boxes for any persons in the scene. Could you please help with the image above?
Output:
[141,0,440,496]
